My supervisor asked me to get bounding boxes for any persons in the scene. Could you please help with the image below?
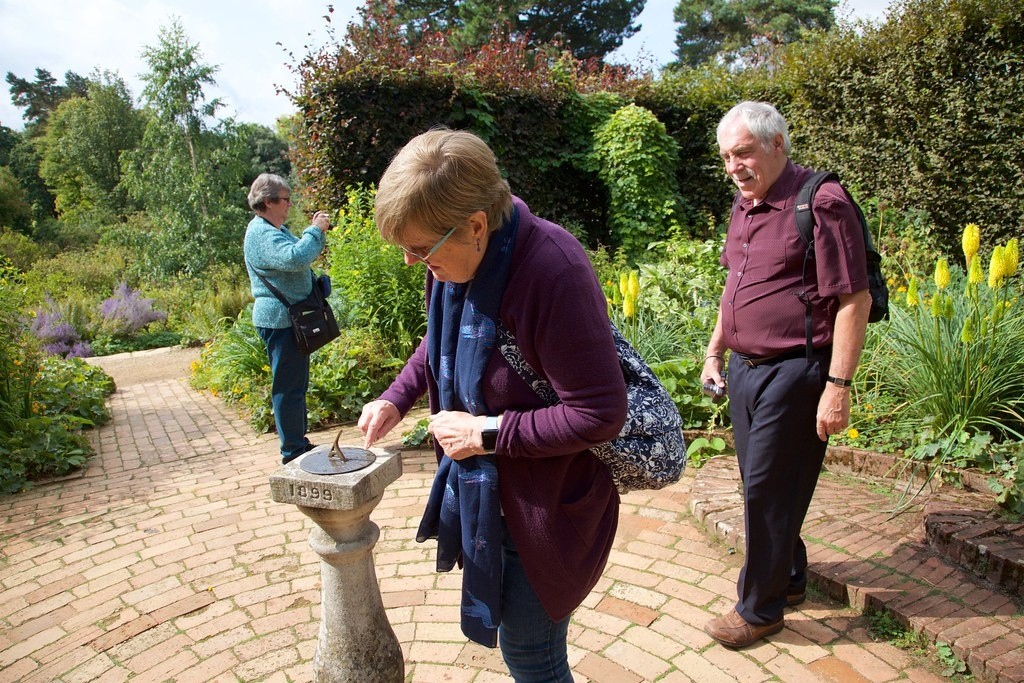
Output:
[355,128,629,683]
[244,173,330,465]
[700,100,873,649]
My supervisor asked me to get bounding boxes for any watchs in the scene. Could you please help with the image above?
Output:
[481,416,500,451]
[827,376,852,387]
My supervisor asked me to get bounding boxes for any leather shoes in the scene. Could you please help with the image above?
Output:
[704,608,785,648]
[785,592,806,606]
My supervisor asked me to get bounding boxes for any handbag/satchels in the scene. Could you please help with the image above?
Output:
[288,284,342,354]
[554,318,688,494]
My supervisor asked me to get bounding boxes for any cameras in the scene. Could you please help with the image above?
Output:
[703,369,727,403]
[323,214,333,230]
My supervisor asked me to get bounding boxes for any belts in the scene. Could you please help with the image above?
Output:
[739,352,796,367]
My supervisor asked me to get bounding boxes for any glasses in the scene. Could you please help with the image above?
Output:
[400,228,455,264]
[280,197,291,203]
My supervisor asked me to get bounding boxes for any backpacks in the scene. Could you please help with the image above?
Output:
[794,170,890,323]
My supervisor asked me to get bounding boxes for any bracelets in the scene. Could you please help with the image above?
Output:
[467,415,496,456]
[704,356,725,362]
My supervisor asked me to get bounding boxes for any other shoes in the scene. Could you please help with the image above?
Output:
[282,444,316,465]
